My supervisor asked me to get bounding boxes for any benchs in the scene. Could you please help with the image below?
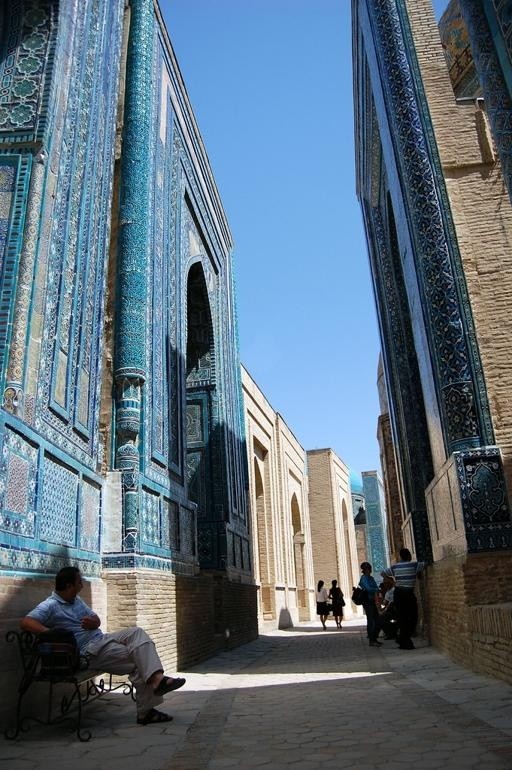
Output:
[4,629,138,742]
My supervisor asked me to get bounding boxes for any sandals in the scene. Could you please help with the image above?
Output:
[155,675,186,696]
[136,709,173,727]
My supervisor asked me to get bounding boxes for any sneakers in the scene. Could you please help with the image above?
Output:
[369,634,415,649]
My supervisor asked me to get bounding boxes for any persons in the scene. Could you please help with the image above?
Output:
[381,548,433,649]
[328,579,346,629]
[315,581,329,631]
[18,566,186,726]
[375,577,399,641]
[359,562,384,648]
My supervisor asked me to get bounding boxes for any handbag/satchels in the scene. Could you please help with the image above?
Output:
[327,603,333,611]
[352,587,364,605]
